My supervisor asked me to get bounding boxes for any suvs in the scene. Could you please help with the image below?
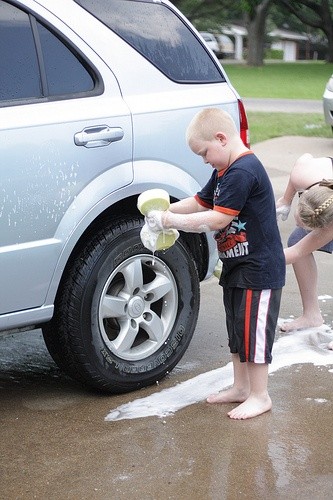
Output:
[199,31,236,60]
[0,0,251,396]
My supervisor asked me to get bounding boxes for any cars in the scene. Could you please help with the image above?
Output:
[322,72,333,133]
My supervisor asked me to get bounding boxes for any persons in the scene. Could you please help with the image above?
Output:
[276,152,333,351]
[145,106,285,420]
